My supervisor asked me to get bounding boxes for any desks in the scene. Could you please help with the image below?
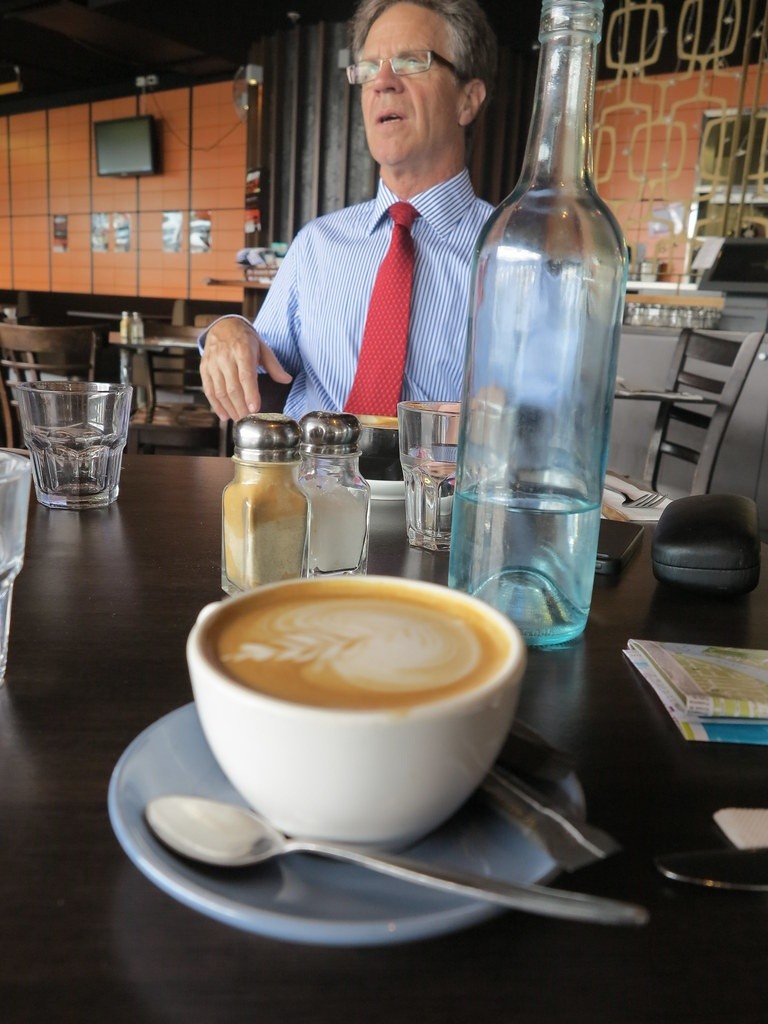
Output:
[105,325,222,386]
[0,451,768,1024]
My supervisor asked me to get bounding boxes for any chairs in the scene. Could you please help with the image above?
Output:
[1,323,110,449]
[643,329,765,495]
[125,320,231,457]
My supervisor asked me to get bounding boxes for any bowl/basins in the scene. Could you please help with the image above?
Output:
[185,572,528,855]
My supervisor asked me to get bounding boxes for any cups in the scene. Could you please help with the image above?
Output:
[0,449,34,685]
[15,379,136,511]
[641,262,659,281]
[623,301,723,328]
[396,399,462,552]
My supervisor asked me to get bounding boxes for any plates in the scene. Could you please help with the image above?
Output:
[107,699,588,948]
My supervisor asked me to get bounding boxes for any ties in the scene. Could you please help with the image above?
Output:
[343,201,422,418]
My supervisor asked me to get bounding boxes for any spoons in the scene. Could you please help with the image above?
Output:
[143,794,650,930]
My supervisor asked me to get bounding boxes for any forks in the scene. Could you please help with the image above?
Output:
[603,482,668,509]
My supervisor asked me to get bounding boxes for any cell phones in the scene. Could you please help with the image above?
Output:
[596,518,644,573]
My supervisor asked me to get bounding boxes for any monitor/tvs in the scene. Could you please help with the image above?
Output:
[94,114,156,177]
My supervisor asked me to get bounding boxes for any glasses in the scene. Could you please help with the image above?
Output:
[347,48,475,85]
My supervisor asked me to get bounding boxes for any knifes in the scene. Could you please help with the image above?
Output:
[657,844,768,893]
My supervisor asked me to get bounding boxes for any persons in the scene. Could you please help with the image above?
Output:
[198,0,585,467]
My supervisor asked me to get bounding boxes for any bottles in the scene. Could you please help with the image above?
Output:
[449,0,629,650]
[121,311,145,340]
[221,410,311,597]
[298,411,371,577]
